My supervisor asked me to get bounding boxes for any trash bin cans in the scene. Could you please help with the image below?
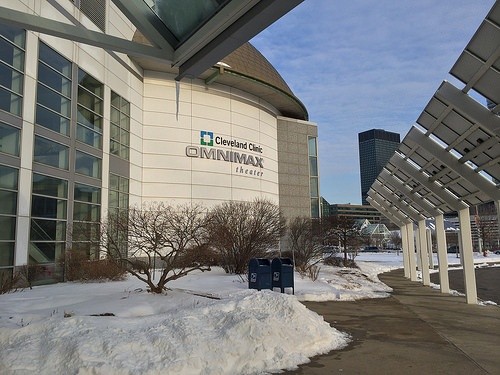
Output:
[271,257,295,296]
[248,258,272,292]
